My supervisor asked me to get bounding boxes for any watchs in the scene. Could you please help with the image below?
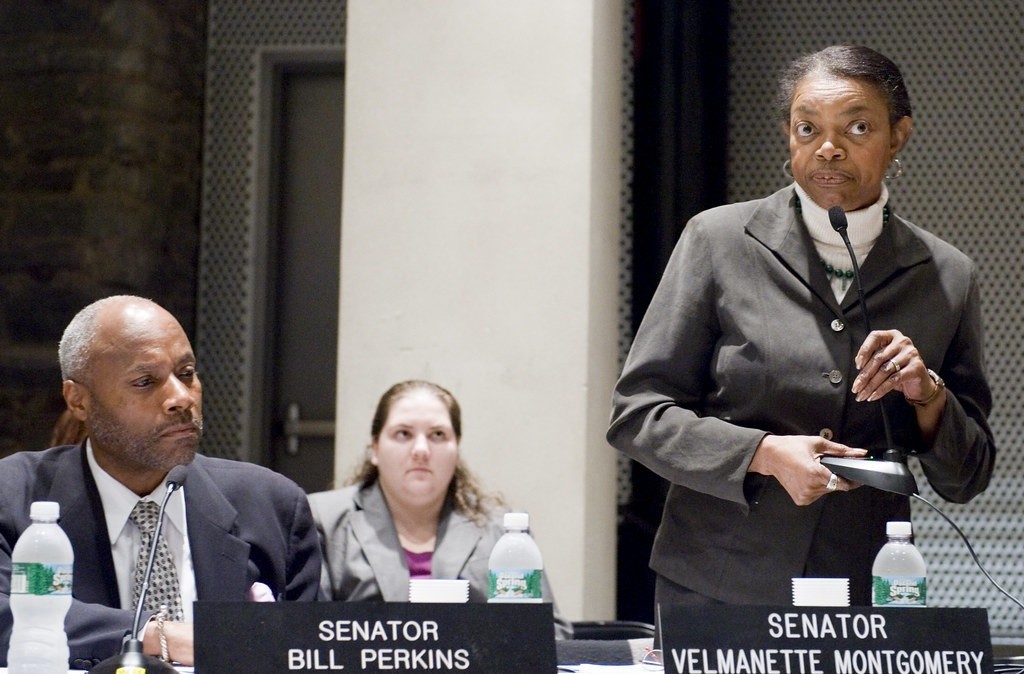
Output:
[905,368,945,408]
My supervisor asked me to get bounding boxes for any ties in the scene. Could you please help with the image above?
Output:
[128,501,181,630]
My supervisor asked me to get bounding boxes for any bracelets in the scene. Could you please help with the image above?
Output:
[157,617,171,662]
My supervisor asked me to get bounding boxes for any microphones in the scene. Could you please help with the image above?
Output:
[88,464,189,674]
[820,205,921,496]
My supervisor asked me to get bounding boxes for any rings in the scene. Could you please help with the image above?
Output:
[890,359,900,371]
[826,474,837,491]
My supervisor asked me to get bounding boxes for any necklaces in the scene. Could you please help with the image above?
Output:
[795,198,890,278]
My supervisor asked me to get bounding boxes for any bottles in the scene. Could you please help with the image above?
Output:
[872,521,927,607]
[486,512,544,603]
[8,500,74,674]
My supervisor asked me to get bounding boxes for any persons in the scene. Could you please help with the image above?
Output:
[306,381,575,644]
[609,44,997,649]
[0,295,322,667]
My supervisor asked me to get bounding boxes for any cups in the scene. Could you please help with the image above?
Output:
[791,578,851,607]
[410,578,471,604]
